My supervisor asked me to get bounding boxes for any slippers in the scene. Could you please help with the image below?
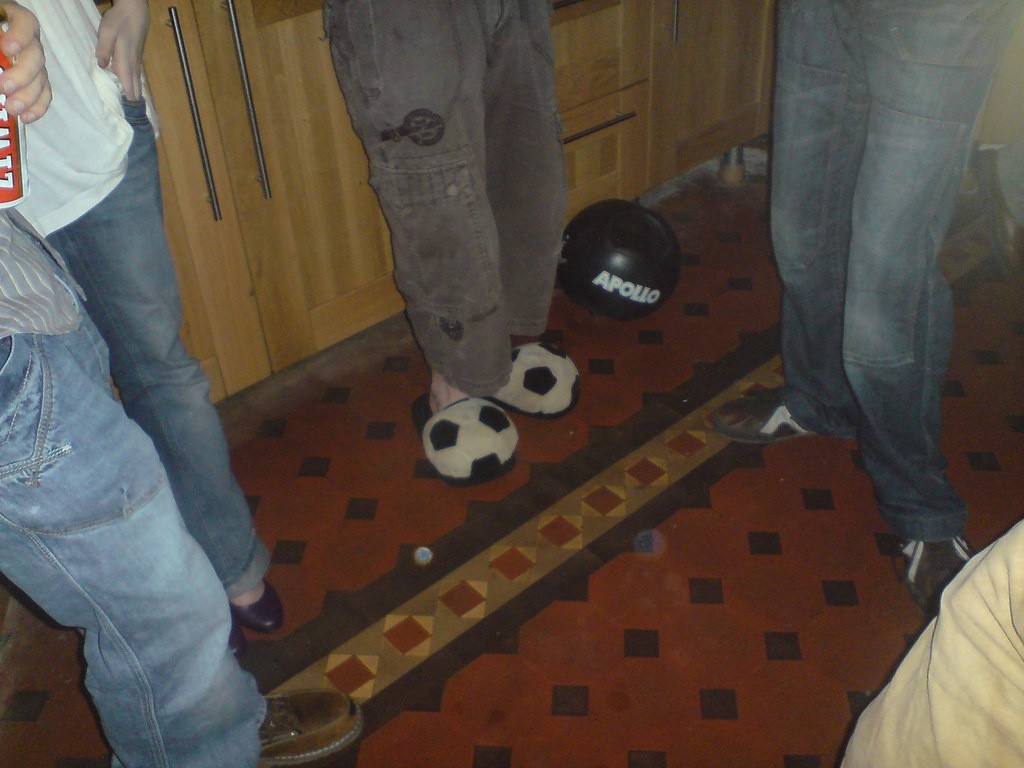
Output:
[483,340,581,420]
[411,393,519,488]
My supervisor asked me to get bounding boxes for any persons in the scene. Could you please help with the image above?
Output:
[707,1,1024,619]
[0,0,358,768]
[320,0,583,482]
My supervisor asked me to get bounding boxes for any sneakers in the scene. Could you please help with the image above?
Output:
[710,388,820,445]
[901,535,977,613]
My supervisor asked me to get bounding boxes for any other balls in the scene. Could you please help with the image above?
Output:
[556,198,682,320]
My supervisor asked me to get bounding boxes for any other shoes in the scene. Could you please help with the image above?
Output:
[259,689,364,765]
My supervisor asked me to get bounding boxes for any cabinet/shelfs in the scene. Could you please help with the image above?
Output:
[92,1,782,409]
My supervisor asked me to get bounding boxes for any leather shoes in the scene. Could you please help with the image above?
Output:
[228,614,248,658]
[230,580,284,634]
